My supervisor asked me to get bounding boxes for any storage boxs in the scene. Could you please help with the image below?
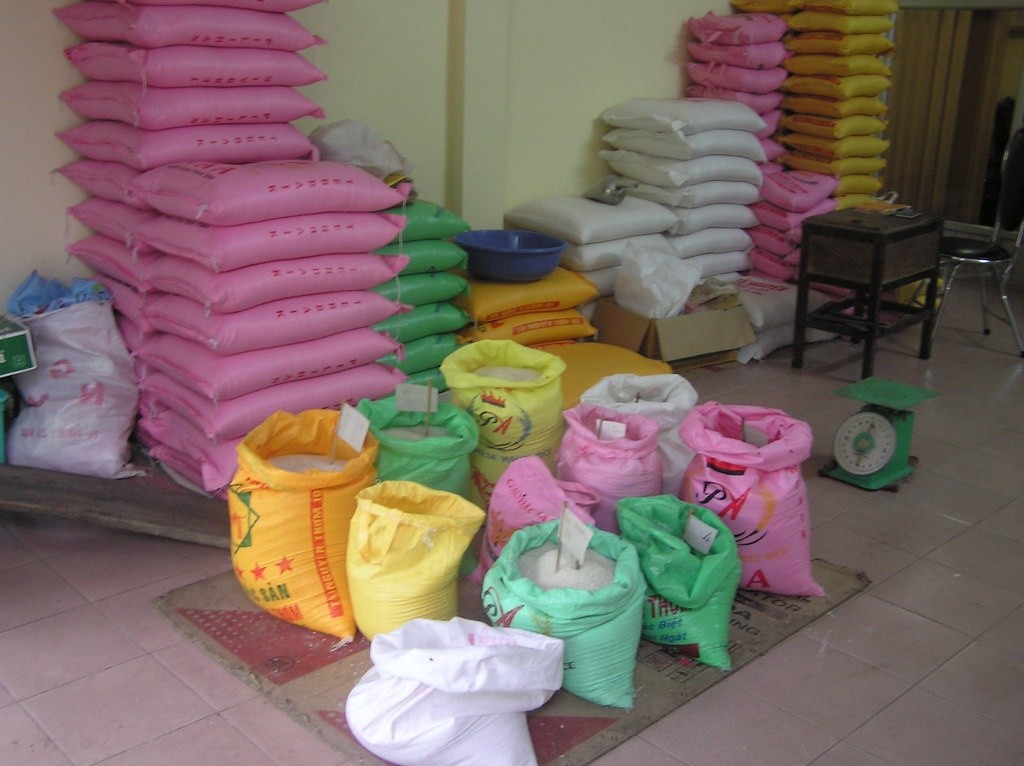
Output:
[584,294,757,372]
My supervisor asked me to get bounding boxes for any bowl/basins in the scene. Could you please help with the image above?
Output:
[453,229,567,280]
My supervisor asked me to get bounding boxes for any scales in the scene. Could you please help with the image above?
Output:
[816,376,939,493]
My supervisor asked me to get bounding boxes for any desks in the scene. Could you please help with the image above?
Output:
[792,203,947,384]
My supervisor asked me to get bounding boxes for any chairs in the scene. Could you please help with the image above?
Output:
[897,128,1024,358]
